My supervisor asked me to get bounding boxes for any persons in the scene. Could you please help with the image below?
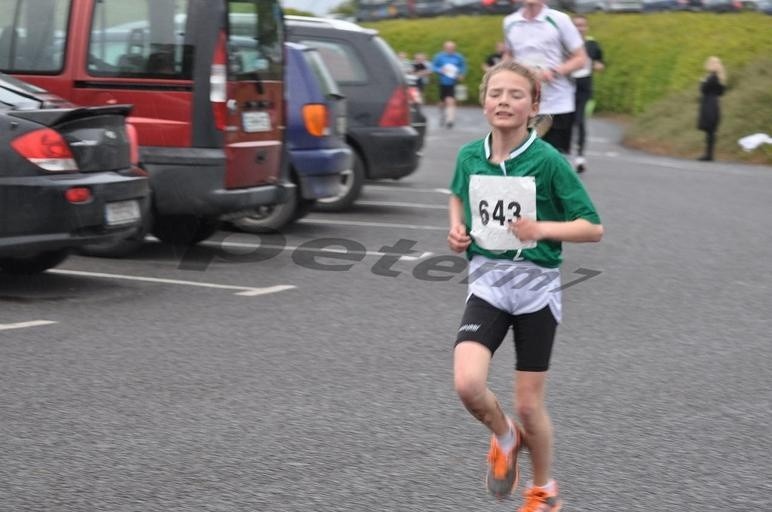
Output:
[695,56,726,160]
[400,40,468,130]
[481,42,503,72]
[498,0,588,157]
[572,14,605,172]
[447,63,603,512]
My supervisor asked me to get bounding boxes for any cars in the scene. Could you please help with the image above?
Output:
[0,72,155,276]
[49,14,428,212]
[41,30,356,233]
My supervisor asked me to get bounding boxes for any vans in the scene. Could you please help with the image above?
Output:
[1,0,298,257]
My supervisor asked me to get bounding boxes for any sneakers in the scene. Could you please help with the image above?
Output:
[486,421,522,497]
[517,483,562,512]
[576,156,586,173]
